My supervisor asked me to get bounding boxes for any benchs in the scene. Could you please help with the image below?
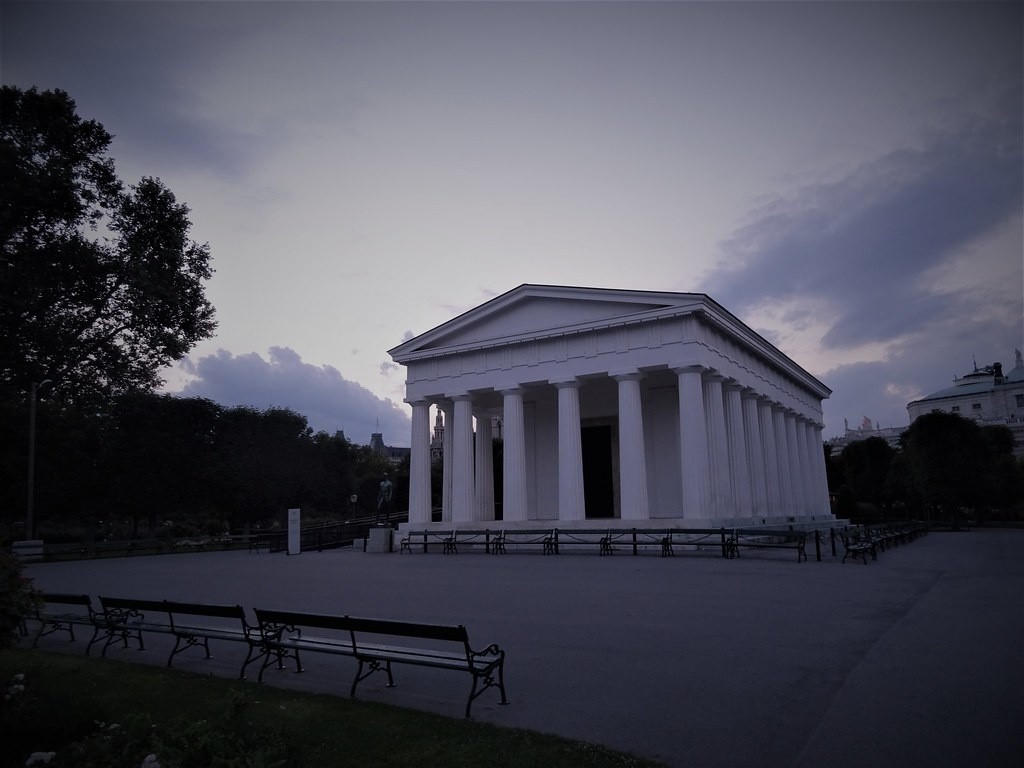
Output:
[604,527,670,557]
[664,527,734,557]
[842,520,929,565]
[170,535,204,553]
[46,543,87,562]
[448,529,503,556]
[98,595,281,679]
[400,529,454,555]
[226,534,248,550]
[251,607,510,724]
[496,528,554,556]
[729,528,807,564]
[133,538,167,558]
[204,535,226,551]
[20,592,128,656]
[97,540,134,559]
[548,527,610,556]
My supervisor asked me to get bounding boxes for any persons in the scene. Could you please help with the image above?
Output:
[370,473,392,527]
